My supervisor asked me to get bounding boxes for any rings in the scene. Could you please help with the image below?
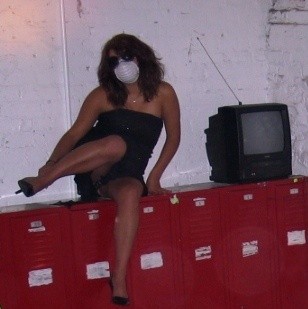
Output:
[160,189,163,192]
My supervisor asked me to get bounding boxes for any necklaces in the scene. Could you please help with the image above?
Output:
[133,98,137,103]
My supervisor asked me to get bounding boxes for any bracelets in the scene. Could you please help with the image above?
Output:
[46,159,56,165]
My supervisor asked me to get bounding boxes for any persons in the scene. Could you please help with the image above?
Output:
[15,34,181,303]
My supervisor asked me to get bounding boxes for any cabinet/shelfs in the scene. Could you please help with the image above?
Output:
[0,173,308,309]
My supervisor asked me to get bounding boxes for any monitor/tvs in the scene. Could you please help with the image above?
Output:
[204,103,291,183]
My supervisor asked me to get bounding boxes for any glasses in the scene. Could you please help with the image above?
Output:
[106,49,137,69]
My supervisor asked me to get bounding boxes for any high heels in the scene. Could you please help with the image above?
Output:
[15,180,34,197]
[110,274,129,304]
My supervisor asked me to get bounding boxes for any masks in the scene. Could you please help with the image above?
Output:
[113,59,140,84]
